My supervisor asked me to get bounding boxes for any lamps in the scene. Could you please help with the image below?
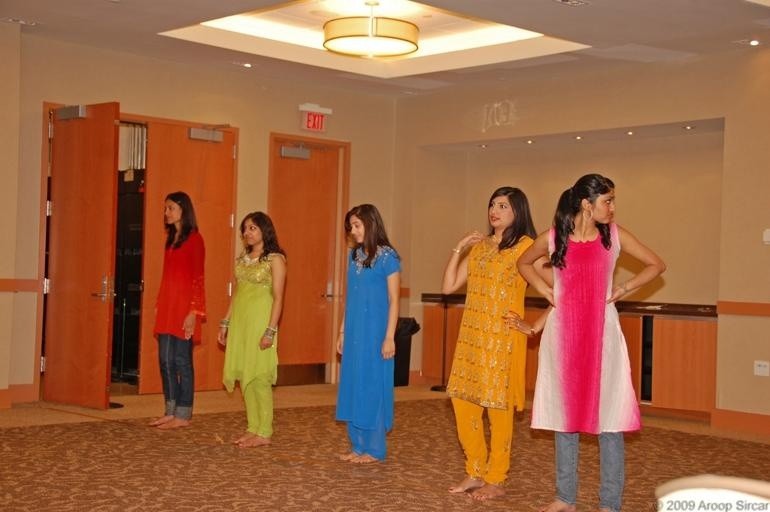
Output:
[323,0,422,63]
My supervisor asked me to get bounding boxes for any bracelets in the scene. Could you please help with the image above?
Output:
[531,324,534,335]
[219,319,230,328]
[264,327,277,340]
[619,282,627,292]
[340,332,344,333]
[452,248,463,254]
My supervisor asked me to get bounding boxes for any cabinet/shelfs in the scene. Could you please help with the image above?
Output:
[418,294,718,423]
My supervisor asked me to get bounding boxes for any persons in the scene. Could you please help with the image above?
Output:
[217,211,288,447]
[441,187,554,501]
[336,204,401,464]
[516,174,667,512]
[148,191,207,430]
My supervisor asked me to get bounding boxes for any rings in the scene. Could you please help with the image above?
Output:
[518,323,519,327]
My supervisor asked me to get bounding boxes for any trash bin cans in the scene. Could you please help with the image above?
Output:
[393,317,420,386]
